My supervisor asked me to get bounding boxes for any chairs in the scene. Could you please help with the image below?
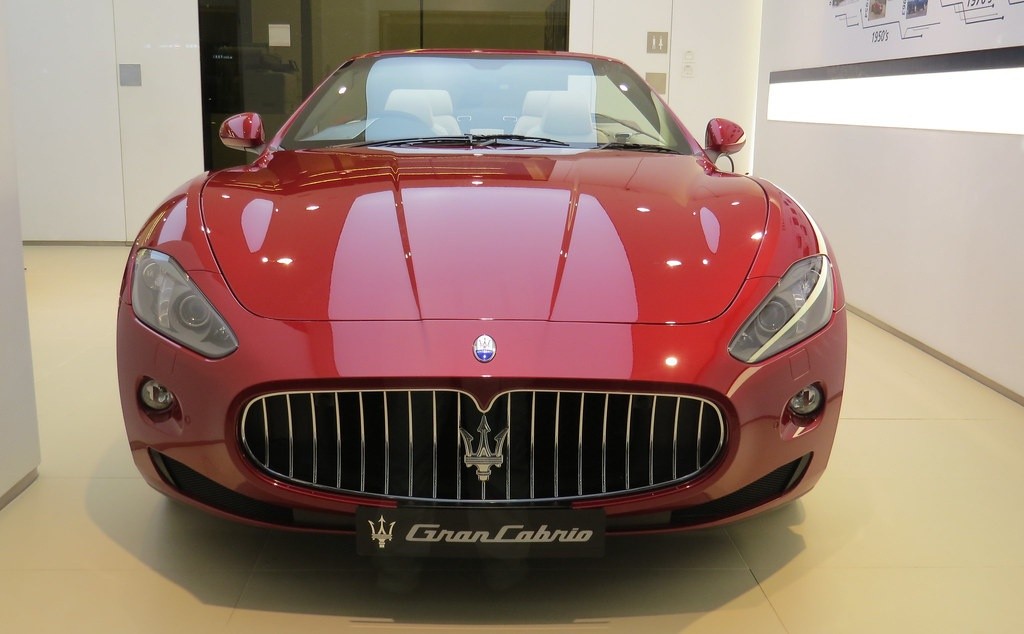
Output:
[390,98,447,136]
[524,96,609,143]
[391,89,462,135]
[514,91,569,136]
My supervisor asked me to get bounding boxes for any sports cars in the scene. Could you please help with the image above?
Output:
[115,47,850,562]
